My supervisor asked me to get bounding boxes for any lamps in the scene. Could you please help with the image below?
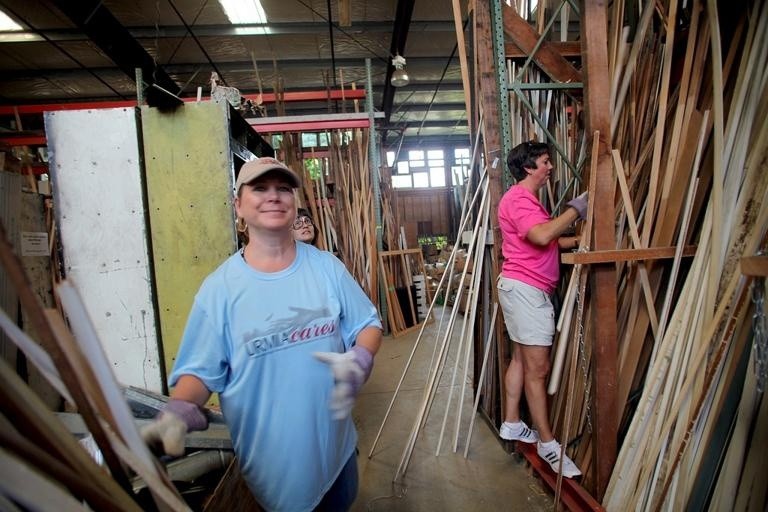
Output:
[390,47,409,88]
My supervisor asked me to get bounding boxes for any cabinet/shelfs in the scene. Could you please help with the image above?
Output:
[43,98,277,413]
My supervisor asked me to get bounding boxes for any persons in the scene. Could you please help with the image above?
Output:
[294,208,319,246]
[140,154,388,511]
[493,141,588,479]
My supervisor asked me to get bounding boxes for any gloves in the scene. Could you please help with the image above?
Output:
[138,398,208,457]
[315,344,373,422]
[568,191,589,218]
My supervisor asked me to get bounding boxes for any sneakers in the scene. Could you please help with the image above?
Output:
[537,439,583,480]
[500,419,540,443]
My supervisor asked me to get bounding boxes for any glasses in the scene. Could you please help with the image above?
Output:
[292,217,314,230]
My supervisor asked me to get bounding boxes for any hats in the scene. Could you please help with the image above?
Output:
[236,158,301,196]
